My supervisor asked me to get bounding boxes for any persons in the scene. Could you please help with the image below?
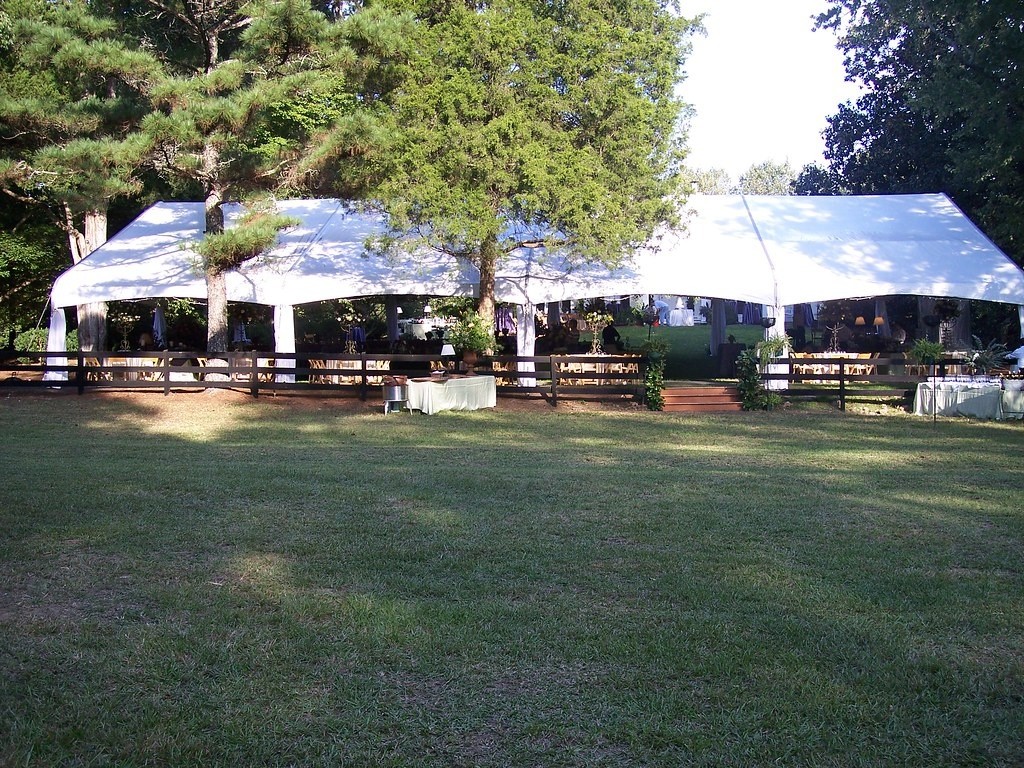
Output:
[892,321,909,353]
[602,323,621,354]
[836,320,851,352]
[566,319,580,346]
[1003,338,1024,374]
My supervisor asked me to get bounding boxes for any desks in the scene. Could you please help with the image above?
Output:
[407,376,496,415]
[913,377,1024,421]
[717,343,746,379]
[102,358,390,384]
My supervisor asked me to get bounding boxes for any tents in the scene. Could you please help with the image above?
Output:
[42,195,1024,389]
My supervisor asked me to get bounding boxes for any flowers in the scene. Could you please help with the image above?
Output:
[577,299,614,325]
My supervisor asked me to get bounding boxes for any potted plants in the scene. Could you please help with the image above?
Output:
[442,307,505,375]
[964,333,1012,375]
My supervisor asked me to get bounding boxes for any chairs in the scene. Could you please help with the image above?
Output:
[902,352,962,375]
[86,358,332,385]
[791,353,880,383]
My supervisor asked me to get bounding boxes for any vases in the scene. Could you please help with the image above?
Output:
[586,322,606,355]
[1010,365,1019,375]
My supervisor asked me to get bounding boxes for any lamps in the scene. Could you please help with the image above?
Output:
[441,344,455,377]
